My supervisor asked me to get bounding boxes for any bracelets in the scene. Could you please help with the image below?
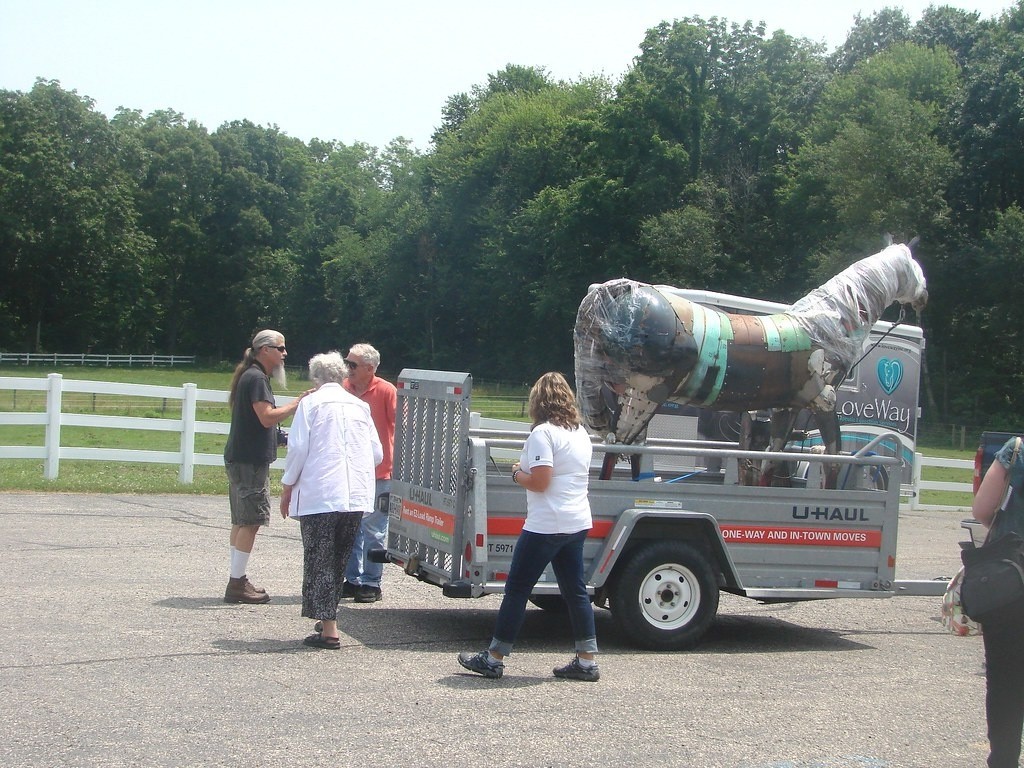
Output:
[512,469,521,483]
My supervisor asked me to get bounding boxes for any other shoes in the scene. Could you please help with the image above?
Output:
[304,633,340,649]
[225,575,270,605]
[247,579,265,593]
[314,621,323,632]
[355,585,382,603]
[340,581,360,598]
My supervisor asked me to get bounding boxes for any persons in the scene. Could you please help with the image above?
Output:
[972,436,1024,768]
[223,332,316,604]
[279,354,385,650]
[341,344,408,604]
[456,371,601,682]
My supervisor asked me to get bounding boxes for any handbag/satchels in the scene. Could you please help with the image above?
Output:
[960,532,1024,623]
[942,565,984,637]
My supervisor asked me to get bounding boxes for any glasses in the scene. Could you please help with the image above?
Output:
[268,345,287,352]
[343,358,369,369]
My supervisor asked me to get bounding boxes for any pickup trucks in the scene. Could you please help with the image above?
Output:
[960,431,1024,548]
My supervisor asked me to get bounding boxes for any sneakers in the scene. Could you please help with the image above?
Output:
[457,652,506,679]
[553,653,600,682]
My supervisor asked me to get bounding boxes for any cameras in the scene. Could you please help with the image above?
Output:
[275,430,288,445]
